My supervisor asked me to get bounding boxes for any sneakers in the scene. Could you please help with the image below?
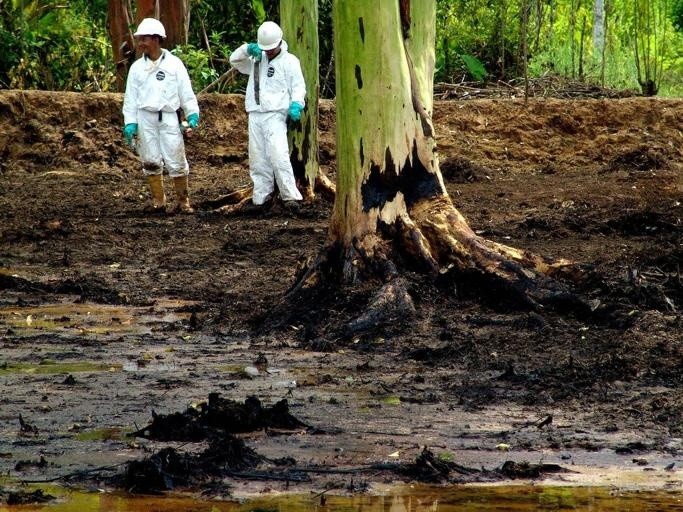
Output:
[284,200,302,209]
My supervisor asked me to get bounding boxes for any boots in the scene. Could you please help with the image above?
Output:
[144,175,166,213]
[173,176,194,213]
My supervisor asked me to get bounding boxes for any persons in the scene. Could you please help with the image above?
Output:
[229,21,306,208]
[123,18,199,214]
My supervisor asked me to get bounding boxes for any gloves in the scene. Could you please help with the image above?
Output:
[123,123,139,144]
[186,113,200,128]
[247,43,262,61]
[288,101,303,122]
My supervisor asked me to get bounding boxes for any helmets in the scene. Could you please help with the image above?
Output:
[133,18,167,39]
[257,21,283,51]
